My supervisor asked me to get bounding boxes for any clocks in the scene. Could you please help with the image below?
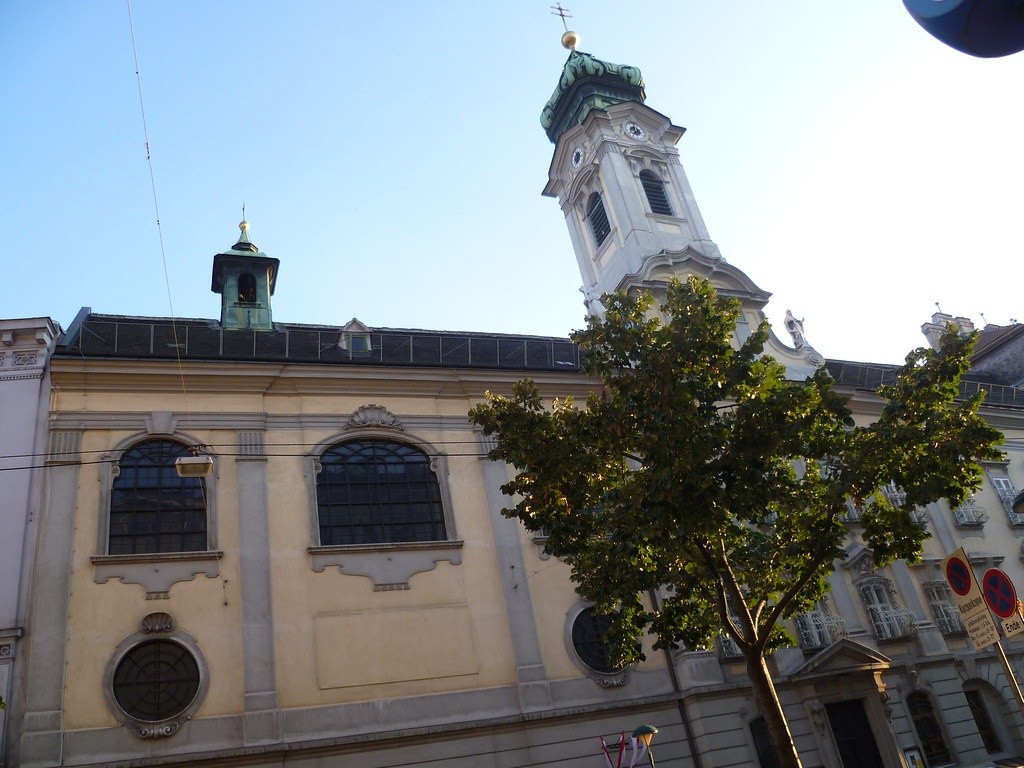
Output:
[572,147,584,168]
[624,123,646,138]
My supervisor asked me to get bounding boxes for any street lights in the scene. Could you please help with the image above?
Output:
[632,725,658,768]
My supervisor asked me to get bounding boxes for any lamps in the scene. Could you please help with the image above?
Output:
[174,451,214,477]
